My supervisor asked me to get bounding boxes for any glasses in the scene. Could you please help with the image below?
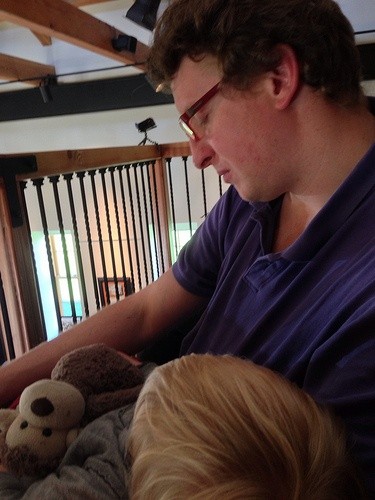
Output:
[178,70,235,143]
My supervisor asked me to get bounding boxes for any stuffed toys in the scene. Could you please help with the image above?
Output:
[0,342,146,479]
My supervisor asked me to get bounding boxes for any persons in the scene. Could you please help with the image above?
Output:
[126,352,352,500]
[0,0,375,500]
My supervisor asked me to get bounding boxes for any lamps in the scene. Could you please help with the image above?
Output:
[144,73,166,93]
[135,117,159,146]
[38,73,57,103]
[125,0,161,31]
[111,34,138,54]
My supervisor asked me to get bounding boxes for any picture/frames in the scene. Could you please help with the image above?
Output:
[98,277,131,309]
